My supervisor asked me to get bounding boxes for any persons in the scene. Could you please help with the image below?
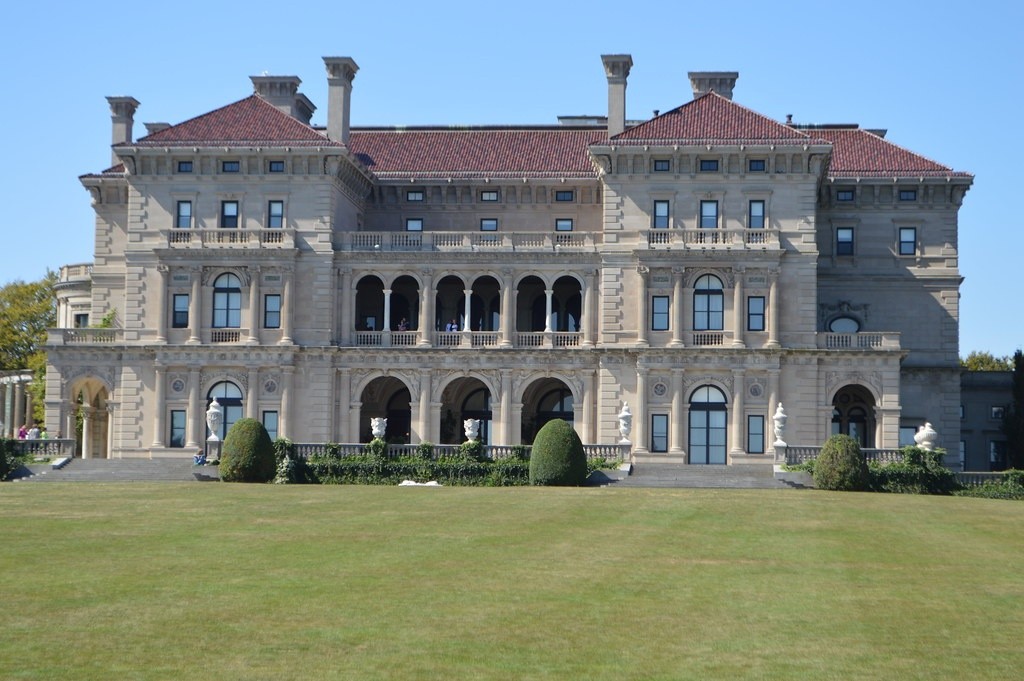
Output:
[194,449,206,466]
[18,424,62,451]
